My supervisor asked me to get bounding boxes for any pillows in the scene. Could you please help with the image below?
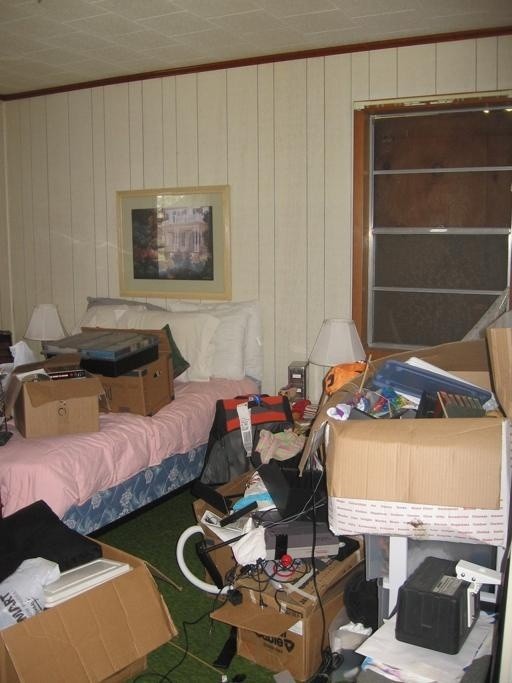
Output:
[83,295,264,383]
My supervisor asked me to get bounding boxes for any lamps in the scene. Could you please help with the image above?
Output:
[25,303,64,355]
[312,320,361,370]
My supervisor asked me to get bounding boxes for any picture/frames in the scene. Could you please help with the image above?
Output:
[115,186,235,307]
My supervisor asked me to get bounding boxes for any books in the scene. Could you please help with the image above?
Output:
[260,519,341,561]
[354,611,496,682]
[35,556,134,610]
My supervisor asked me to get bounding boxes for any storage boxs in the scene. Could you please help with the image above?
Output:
[0,527,181,683]
[194,472,359,666]
[0,366,110,436]
[59,324,176,416]
[326,328,509,547]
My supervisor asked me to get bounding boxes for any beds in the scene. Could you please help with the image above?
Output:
[2,376,271,534]
